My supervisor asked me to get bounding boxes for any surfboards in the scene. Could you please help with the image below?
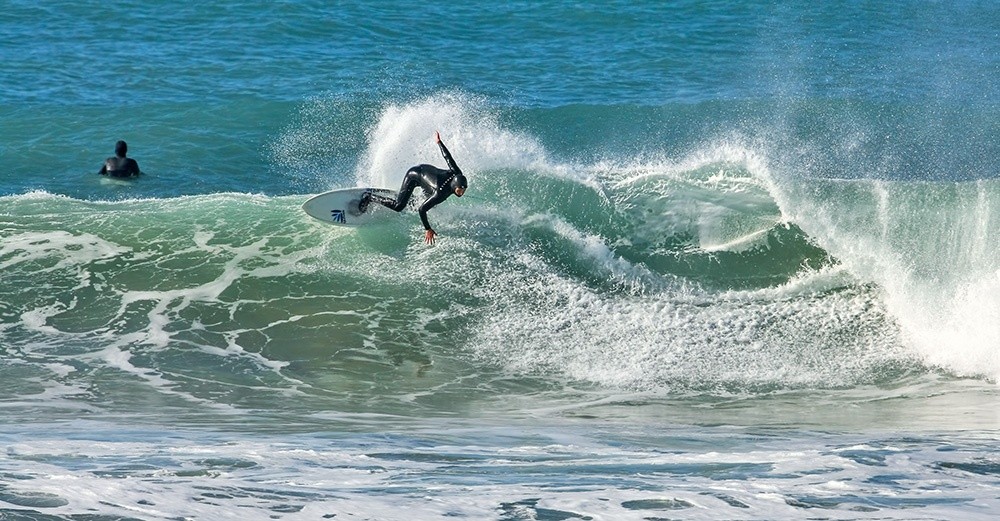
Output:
[301,187,418,226]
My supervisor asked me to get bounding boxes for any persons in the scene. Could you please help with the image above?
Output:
[359,130,467,245]
[101,140,139,178]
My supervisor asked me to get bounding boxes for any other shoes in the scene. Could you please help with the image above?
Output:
[359,192,372,214]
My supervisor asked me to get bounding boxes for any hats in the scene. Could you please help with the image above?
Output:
[115,141,127,156]
